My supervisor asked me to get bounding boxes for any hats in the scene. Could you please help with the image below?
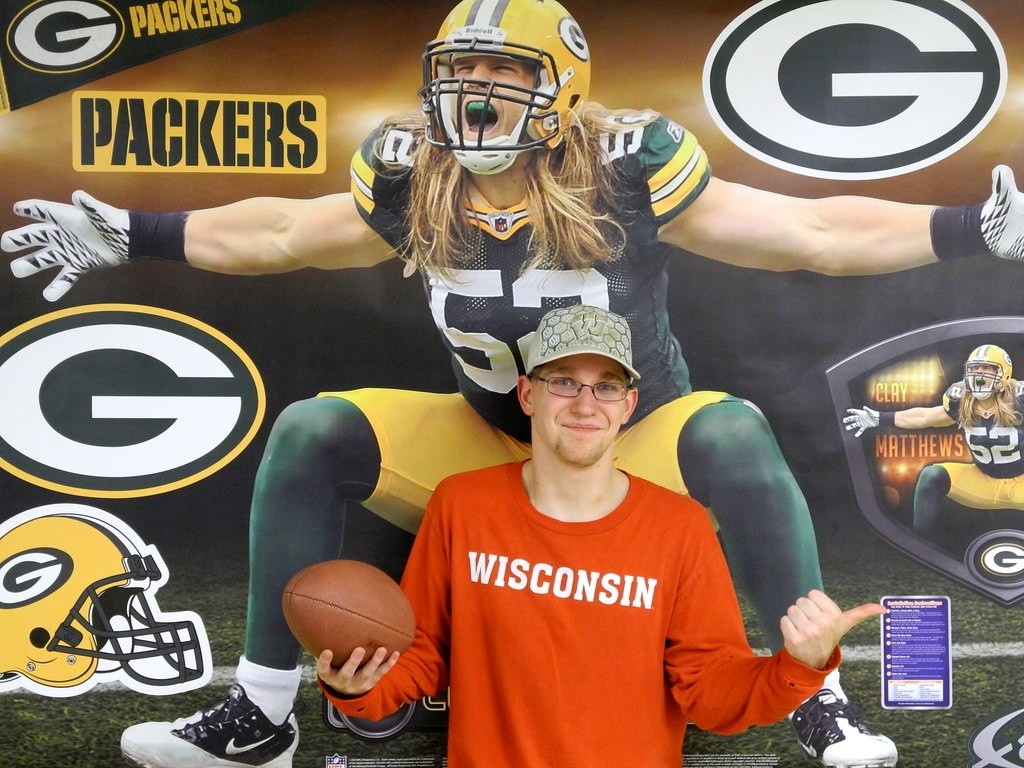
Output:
[527,304,641,386]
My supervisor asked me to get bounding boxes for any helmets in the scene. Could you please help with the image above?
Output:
[422,0,591,152]
[963,344,1012,391]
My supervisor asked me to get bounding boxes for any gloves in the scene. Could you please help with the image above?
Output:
[843,406,896,437]
[1,191,192,302]
[931,165,1024,264]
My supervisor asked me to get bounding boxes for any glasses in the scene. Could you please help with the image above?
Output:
[528,372,630,402]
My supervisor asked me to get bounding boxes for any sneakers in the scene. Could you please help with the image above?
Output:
[788,687,897,768]
[120,682,300,768]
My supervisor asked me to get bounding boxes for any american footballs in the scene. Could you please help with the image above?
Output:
[282,559,417,668]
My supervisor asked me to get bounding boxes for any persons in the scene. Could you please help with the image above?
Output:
[316,306,888,768]
[0,0,1024,768]
[843,345,1024,541]
